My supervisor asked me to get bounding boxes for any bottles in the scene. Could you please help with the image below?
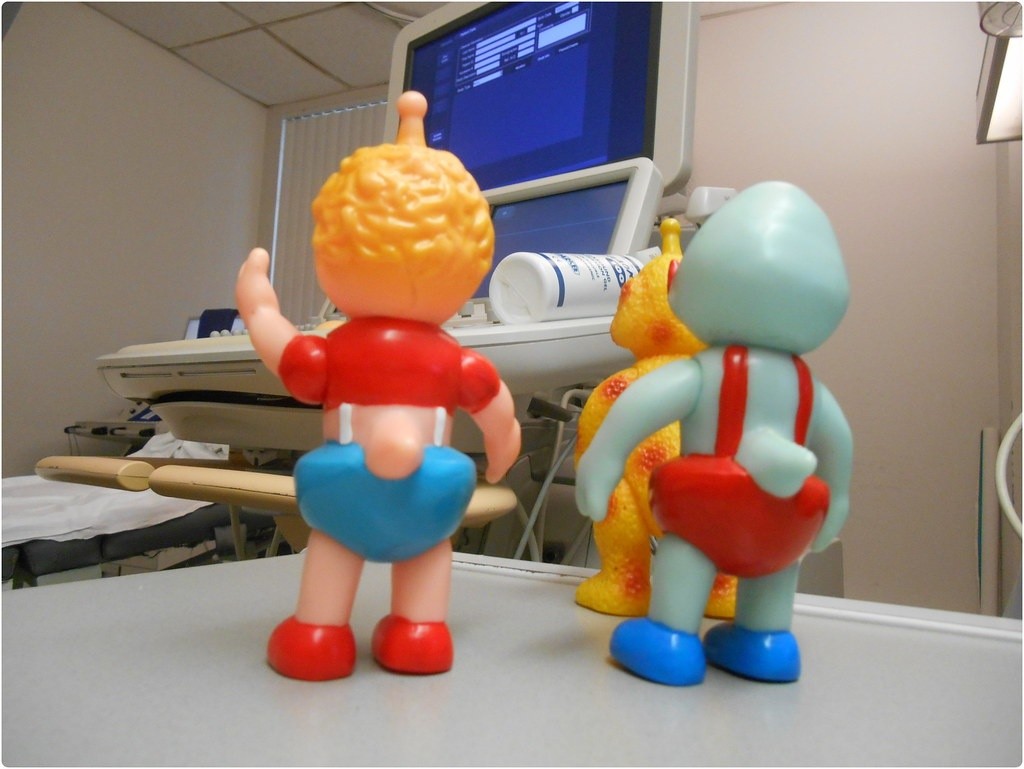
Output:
[487,251,644,326]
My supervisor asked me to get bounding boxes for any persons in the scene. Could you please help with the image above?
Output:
[576,180,852,681]
[233,88,520,681]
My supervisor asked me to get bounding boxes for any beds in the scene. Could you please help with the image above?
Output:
[2,429,277,590]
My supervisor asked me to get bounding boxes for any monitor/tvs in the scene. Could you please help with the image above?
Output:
[383,1,700,195]
[466,156,665,304]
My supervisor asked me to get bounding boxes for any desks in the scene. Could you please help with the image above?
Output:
[2,542,1022,766]
